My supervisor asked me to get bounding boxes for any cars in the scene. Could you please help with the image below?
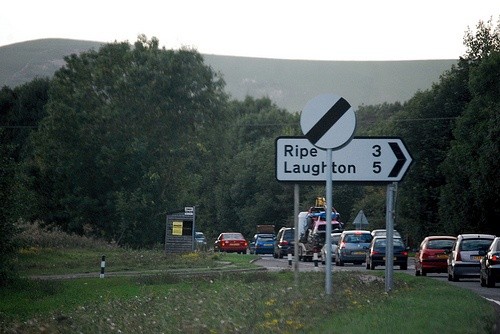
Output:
[194,231,208,245]
[362,236,410,270]
[334,229,374,266]
[444,233,497,282]
[213,231,249,254]
[249,233,278,257]
[412,235,458,277]
[478,236,500,289]
[296,211,403,267]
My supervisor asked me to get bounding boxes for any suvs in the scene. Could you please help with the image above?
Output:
[272,226,295,259]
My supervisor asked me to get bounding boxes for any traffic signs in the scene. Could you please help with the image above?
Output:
[274,134,414,184]
